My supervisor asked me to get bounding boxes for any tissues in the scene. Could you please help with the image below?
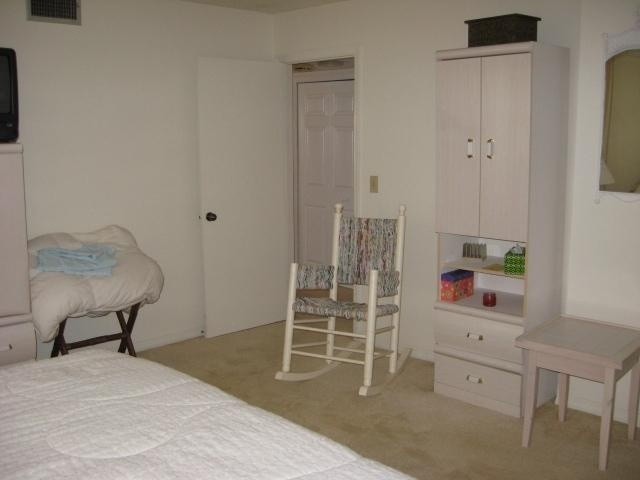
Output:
[503,242,526,276]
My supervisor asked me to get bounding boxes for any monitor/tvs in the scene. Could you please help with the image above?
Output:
[0,46,19,145]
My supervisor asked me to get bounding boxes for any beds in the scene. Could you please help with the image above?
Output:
[1,347,415,479]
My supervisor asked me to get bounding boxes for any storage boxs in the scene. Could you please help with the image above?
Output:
[464,13,542,49]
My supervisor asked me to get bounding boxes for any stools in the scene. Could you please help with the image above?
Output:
[514,314,638,471]
[29,226,165,358]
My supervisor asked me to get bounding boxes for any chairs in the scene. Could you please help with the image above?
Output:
[274,201,415,398]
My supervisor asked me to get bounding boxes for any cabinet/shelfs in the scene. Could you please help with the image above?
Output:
[1,141,36,363]
[433,42,571,420]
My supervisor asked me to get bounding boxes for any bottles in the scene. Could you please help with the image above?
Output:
[483,292,496,307]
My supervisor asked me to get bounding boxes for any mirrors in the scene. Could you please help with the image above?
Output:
[592,23,638,205]
